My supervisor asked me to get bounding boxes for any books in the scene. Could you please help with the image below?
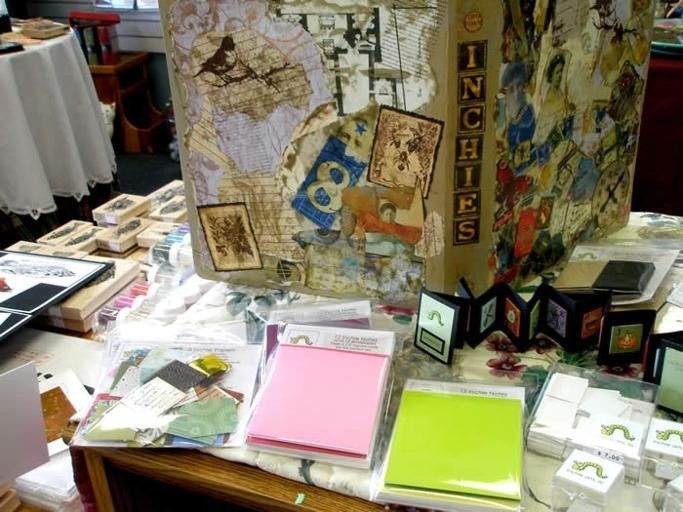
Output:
[591,258,657,297]
[547,259,609,294]
[20,21,72,41]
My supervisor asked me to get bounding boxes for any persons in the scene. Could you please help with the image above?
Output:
[498,61,537,147]
[544,52,568,99]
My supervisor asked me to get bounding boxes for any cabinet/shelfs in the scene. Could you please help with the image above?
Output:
[632,59,682,221]
[86,50,166,155]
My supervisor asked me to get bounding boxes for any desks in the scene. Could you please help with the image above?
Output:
[59,447,389,511]
